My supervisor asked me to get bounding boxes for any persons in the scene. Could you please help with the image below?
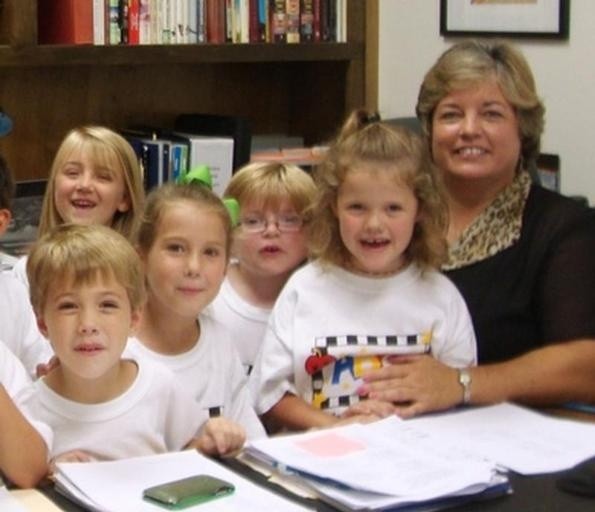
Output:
[195,157,321,375]
[22,221,246,476]
[0,151,61,380]
[0,339,56,488]
[10,126,149,286]
[246,109,479,434]
[120,181,270,446]
[353,39,595,420]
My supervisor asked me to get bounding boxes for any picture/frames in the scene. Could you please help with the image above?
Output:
[437,0,570,42]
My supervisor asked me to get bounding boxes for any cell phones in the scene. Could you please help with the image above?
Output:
[144,476,235,507]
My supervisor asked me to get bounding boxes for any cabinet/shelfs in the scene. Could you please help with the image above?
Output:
[3,2,379,242]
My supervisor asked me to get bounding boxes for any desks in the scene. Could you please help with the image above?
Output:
[39,405,593,511]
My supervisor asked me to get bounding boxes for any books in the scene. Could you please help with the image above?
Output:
[48,448,307,511]
[35,1,351,45]
[118,128,189,194]
[235,401,595,511]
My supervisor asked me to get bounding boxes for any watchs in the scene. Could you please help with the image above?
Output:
[456,367,476,406]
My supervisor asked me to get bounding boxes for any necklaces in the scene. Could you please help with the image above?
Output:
[348,259,410,275]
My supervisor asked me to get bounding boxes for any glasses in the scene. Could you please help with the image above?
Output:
[232,212,311,233]
